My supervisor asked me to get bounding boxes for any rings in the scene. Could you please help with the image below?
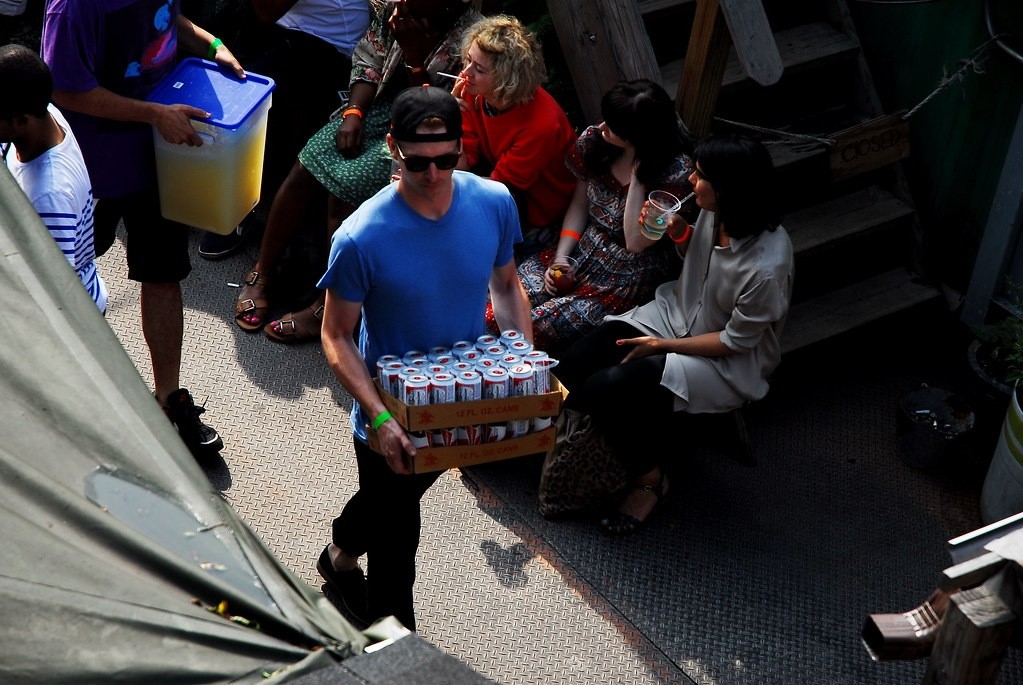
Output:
[394,15,414,26]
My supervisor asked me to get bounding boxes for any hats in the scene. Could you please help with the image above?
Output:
[390,83,464,142]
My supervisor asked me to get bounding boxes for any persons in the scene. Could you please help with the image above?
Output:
[233,0,576,341]
[0,0,246,453]
[181,0,371,259]
[0,44,107,317]
[484,78,794,534]
[315,175,534,631]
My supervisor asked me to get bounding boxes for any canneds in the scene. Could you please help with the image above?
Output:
[376,330,552,449]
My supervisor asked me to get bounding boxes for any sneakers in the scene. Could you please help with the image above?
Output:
[317,543,368,625]
[199,212,254,260]
[152,385,226,457]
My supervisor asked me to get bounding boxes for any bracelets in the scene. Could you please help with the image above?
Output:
[559,230,580,241]
[343,105,363,119]
[371,411,392,430]
[669,224,690,243]
[207,38,221,58]
[403,60,425,73]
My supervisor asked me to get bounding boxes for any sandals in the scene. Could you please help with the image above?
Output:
[601,475,674,535]
[235,264,279,332]
[264,301,322,345]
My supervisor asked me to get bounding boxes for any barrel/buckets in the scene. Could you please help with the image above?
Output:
[899,384,976,471]
[146,56,277,236]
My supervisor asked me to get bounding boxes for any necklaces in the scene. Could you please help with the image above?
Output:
[415,5,453,37]
[485,99,493,118]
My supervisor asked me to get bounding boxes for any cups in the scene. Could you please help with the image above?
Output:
[641,190,681,241]
[548,255,579,296]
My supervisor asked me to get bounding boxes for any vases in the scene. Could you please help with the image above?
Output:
[896,387,975,471]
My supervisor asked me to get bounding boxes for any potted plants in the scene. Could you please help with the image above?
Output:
[966,277,1023,526]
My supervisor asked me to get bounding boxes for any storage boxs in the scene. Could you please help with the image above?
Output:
[146,57,275,237]
[366,371,569,475]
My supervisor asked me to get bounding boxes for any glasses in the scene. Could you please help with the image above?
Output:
[396,144,462,172]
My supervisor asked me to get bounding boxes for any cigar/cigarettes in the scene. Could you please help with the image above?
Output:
[436,72,465,81]
